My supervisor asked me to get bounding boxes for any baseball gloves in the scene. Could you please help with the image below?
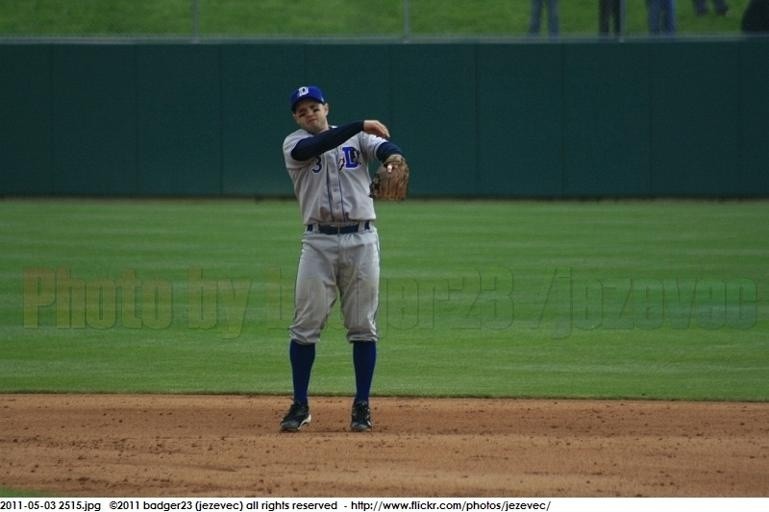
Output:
[368,155,410,201]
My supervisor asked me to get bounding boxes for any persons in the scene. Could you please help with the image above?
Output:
[691,1,728,16]
[526,0,561,39]
[280,85,412,435]
[644,0,678,33]
[595,0,626,40]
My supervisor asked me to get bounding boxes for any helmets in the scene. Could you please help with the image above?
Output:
[290,85,326,107]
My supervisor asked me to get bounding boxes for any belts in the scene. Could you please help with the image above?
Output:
[307,220,371,235]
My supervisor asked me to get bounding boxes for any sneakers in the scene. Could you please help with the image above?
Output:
[280,403,312,431]
[350,403,373,431]
